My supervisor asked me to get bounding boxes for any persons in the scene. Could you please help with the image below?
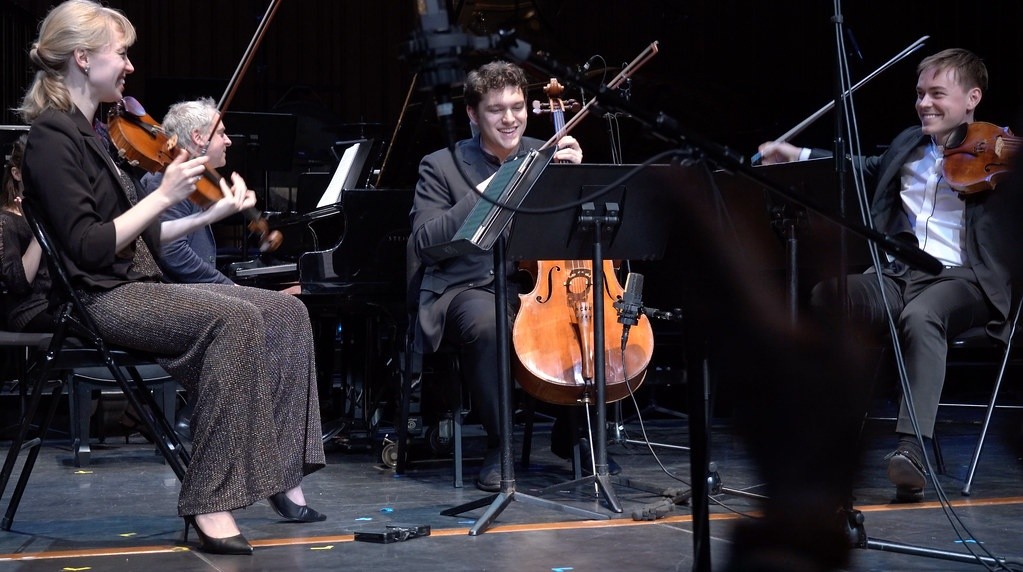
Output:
[408,63,621,491]
[0,135,157,447]
[139,99,302,442]
[18,0,328,553]
[759,48,1023,504]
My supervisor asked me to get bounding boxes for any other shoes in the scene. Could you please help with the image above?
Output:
[884,446,929,502]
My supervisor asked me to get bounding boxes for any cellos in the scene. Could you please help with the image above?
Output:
[510,76,657,497]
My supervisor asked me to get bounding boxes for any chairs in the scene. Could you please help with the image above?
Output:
[847,299,1023,495]
[0,189,193,530]
[395,232,583,487]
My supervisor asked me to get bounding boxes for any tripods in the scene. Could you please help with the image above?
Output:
[437,162,770,535]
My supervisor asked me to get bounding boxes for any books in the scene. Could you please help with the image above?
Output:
[316,143,360,208]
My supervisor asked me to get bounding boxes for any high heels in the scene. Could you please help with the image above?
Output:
[267,492,327,522]
[117,410,154,445]
[183,514,254,554]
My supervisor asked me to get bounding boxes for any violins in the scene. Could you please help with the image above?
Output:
[106,93,287,254]
[942,121,1021,194]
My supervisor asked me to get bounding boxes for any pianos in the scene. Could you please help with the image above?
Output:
[227,137,467,468]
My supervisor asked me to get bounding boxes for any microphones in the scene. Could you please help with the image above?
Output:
[562,58,594,97]
[415,0,458,157]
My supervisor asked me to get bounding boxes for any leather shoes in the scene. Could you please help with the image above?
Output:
[551,436,623,475]
[477,448,503,490]
